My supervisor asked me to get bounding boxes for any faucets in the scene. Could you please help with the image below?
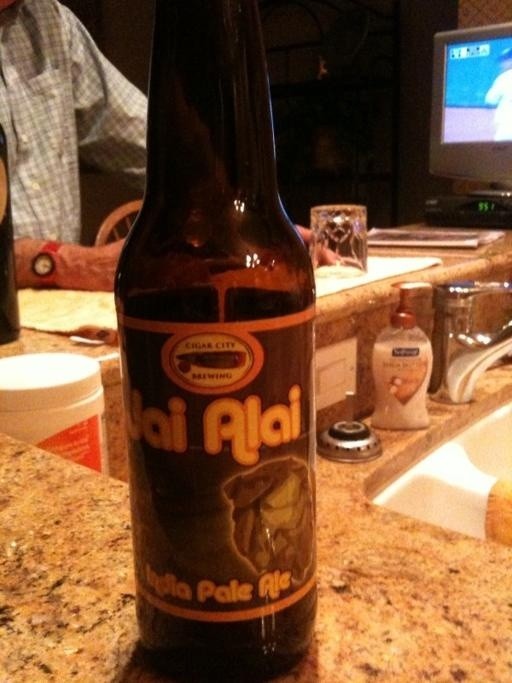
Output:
[445,333,512,402]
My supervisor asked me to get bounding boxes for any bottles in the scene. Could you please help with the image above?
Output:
[113,1,316,677]
[0,351,109,474]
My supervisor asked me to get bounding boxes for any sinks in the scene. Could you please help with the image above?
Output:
[459,405,512,487]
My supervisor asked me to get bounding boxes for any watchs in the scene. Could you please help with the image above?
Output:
[31,239,63,288]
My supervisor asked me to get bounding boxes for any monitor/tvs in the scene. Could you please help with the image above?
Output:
[427,21,512,197]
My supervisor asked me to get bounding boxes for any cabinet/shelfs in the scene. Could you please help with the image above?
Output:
[258,0,402,228]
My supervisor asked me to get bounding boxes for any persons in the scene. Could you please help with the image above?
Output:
[485,47,512,143]
[1,1,338,293]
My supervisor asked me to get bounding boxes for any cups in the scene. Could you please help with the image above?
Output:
[307,204,369,279]
